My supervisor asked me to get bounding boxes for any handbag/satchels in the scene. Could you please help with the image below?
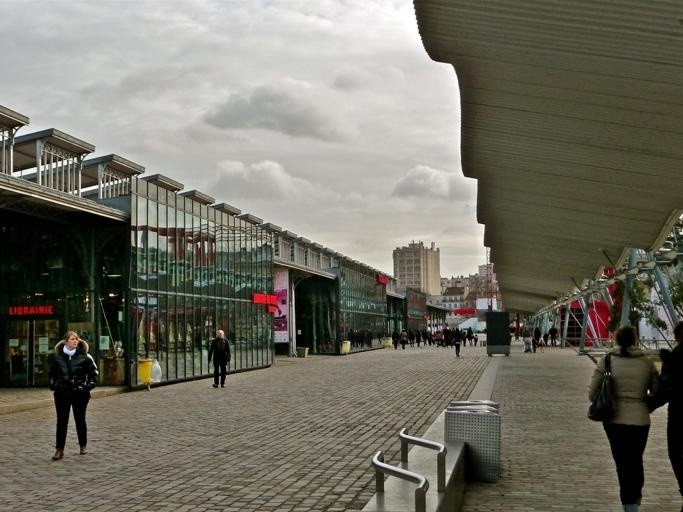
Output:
[587,353,617,421]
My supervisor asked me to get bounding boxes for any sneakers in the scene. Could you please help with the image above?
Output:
[624,505,640,512]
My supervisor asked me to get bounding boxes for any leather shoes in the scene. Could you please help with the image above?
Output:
[213,384,224,388]
[52,450,64,460]
[80,446,88,454]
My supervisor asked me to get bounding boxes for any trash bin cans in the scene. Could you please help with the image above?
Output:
[444,399,501,483]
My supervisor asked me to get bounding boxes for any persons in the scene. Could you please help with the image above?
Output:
[586,325,660,511]
[391,325,487,357]
[208,328,232,389]
[47,329,100,461]
[647,320,683,511]
[339,327,391,350]
[508,324,559,353]
